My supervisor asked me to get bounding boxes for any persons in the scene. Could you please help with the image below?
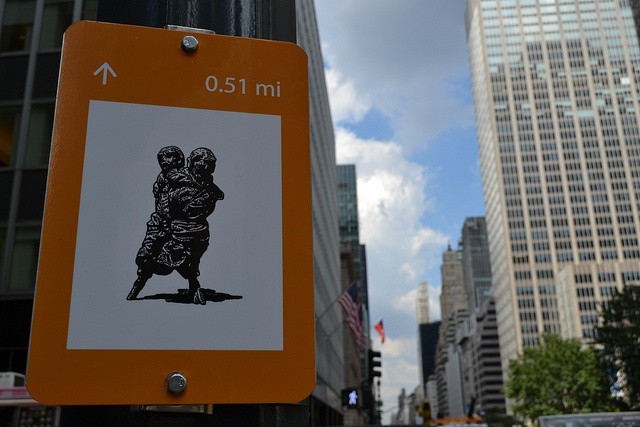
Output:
[127,146,224,305]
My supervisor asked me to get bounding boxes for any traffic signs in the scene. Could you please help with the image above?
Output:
[27,20,316,406]
